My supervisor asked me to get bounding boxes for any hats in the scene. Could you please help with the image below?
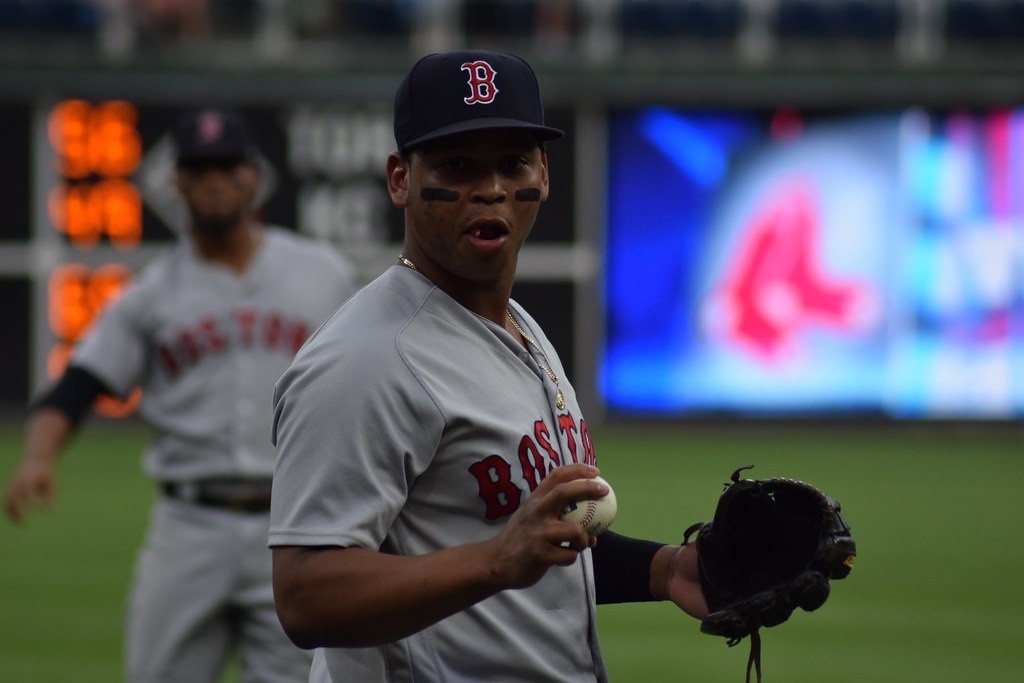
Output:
[174,109,251,165]
[393,49,566,151]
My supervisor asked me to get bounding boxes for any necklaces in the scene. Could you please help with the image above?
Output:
[398,253,565,410]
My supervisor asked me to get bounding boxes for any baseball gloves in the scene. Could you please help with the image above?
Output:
[680,463,860,683]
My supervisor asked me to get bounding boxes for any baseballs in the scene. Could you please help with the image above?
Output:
[558,474,619,536]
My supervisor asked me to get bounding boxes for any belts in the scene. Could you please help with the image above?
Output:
[158,478,276,516]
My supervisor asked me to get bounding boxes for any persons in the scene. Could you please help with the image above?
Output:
[267,51,855,683]
[0,108,356,683]
